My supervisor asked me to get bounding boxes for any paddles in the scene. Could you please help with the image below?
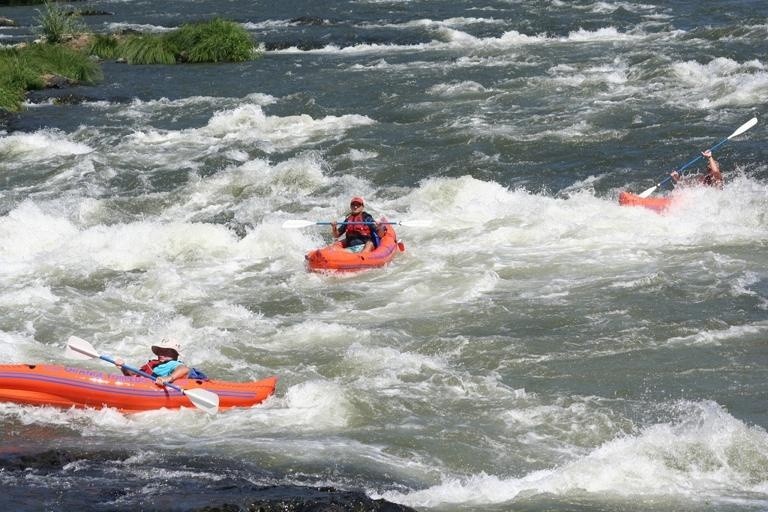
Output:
[66,336,220,415]
[638,118,758,198]
[283,219,437,227]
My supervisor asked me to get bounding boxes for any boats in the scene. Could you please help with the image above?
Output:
[305,217,399,276]
[617,190,688,214]
[0,363,280,415]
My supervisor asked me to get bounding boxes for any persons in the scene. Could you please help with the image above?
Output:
[331,198,381,253]
[670,150,723,189]
[115,345,190,386]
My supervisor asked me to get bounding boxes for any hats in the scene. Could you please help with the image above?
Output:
[151,334,184,355]
[350,197,363,205]
